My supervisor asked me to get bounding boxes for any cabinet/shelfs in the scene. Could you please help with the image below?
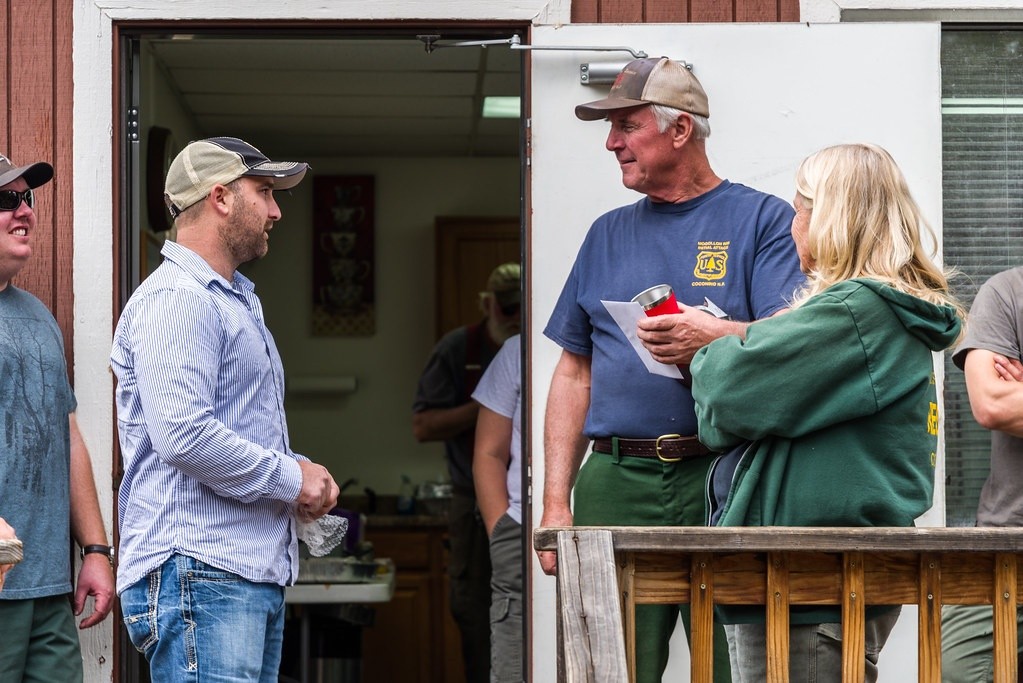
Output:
[434,214,521,352]
[358,494,469,683]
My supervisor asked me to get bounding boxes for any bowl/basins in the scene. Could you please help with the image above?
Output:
[309,558,381,579]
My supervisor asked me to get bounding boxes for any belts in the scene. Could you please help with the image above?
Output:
[593,434,710,462]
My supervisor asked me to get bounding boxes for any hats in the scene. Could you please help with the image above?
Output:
[163,137,307,219]
[488,262,522,317]
[575,55,709,121]
[0,154,54,187]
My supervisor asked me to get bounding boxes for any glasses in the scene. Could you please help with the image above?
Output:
[0,189,35,210]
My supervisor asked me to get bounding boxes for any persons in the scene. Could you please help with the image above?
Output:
[411,263,523,683]
[0,153,116,683]
[535,56,1023,683]
[110,136,340,683]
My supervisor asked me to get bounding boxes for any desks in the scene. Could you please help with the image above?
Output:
[118,553,397,683]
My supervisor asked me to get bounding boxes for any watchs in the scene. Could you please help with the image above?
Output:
[80,544,115,567]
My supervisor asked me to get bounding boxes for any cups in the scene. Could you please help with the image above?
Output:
[631,284,682,317]
